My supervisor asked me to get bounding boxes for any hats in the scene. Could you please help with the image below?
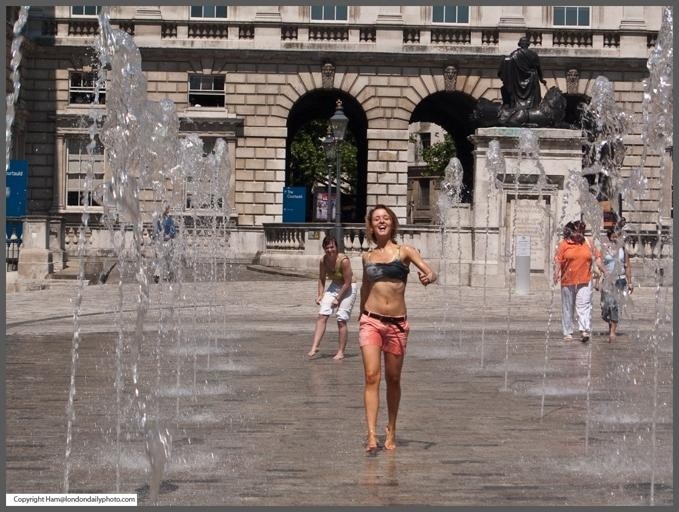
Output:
[563,221,586,238]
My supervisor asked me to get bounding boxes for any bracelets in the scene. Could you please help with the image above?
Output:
[335,296,340,302]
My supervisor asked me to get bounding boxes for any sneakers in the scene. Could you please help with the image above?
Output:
[564,335,572,340]
[580,333,590,342]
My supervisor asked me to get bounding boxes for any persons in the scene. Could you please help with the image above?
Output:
[306,236,357,359]
[554,219,609,342]
[358,203,436,453]
[152,204,176,284]
[595,227,634,338]
[500,37,546,107]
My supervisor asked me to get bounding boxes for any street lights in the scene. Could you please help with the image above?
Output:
[318,98,351,254]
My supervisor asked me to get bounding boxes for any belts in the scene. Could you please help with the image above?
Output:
[363,310,407,333]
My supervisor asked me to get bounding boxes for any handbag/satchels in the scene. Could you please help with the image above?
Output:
[616,279,626,289]
